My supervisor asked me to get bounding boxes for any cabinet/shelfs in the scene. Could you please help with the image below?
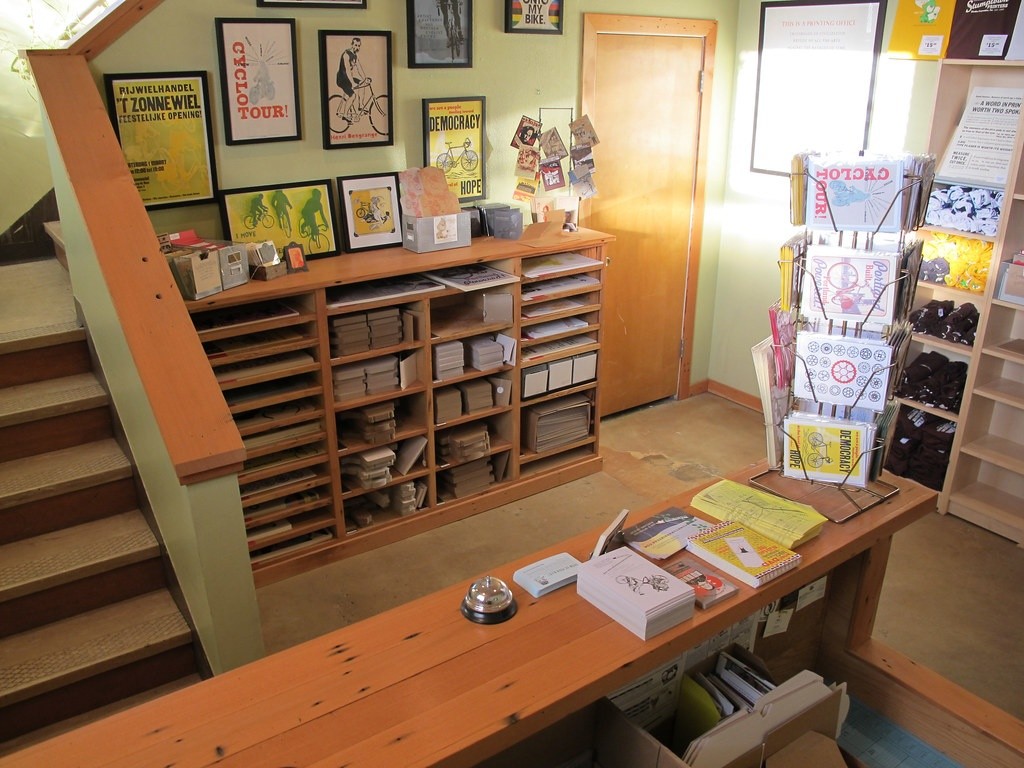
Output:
[0,458,938,768]
[184,222,616,589]
[880,58,1024,547]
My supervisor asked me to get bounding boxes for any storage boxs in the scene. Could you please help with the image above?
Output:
[333,309,504,498]
[249,261,286,281]
[593,643,850,768]
[401,210,471,253]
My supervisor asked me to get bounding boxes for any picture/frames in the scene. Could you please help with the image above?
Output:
[422,96,486,203]
[405,0,473,68]
[214,17,301,145]
[216,179,341,261]
[103,70,218,211]
[336,172,403,253]
[256,0,367,9]
[504,0,564,35]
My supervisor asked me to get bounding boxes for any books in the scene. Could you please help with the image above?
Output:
[661,558,740,610]
[685,520,802,589]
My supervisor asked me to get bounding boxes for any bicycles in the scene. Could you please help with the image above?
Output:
[244,209,274,229]
[356,201,372,218]
[436,2,462,60]
[299,216,330,254]
[278,204,291,238]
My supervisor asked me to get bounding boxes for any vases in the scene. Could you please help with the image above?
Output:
[319,29,393,149]
